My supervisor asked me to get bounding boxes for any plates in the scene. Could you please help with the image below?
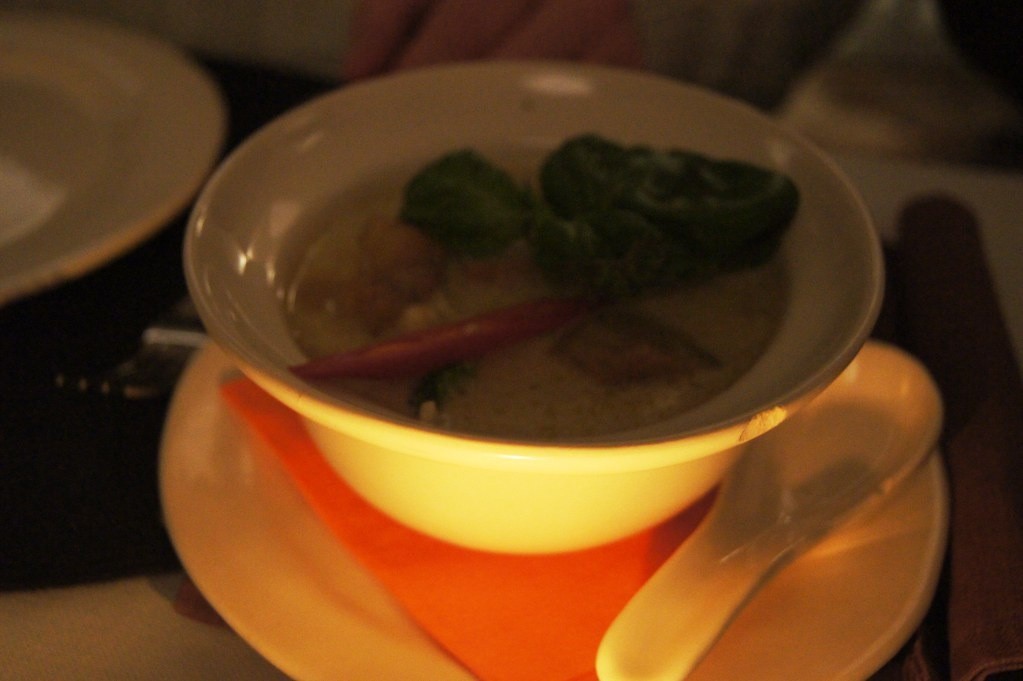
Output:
[155,334,946,681]
[1,9,222,311]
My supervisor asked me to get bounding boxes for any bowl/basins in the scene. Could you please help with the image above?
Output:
[182,55,888,555]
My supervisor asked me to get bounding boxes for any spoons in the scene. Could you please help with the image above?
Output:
[591,343,945,681]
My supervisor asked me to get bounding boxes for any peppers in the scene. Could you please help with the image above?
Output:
[286,295,604,381]
